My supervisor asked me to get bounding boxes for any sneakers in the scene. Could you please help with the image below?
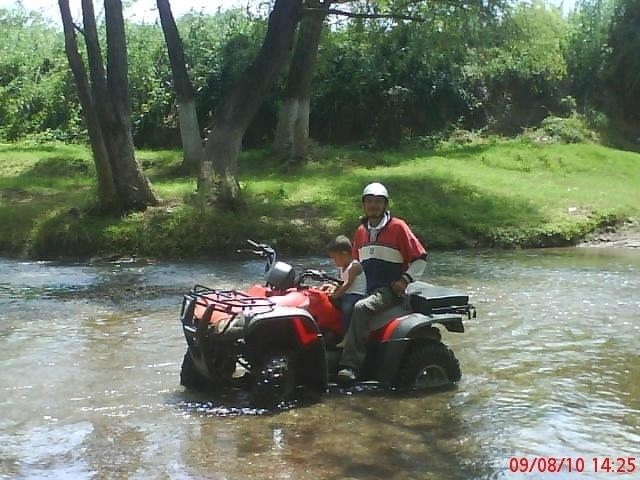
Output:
[338,367,354,382]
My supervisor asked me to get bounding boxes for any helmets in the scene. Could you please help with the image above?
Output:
[361,183,389,203]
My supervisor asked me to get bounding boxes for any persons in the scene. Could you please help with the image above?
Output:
[327,234,368,348]
[332,181,429,383]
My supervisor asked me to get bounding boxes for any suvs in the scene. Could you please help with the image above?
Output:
[180,241,476,409]
